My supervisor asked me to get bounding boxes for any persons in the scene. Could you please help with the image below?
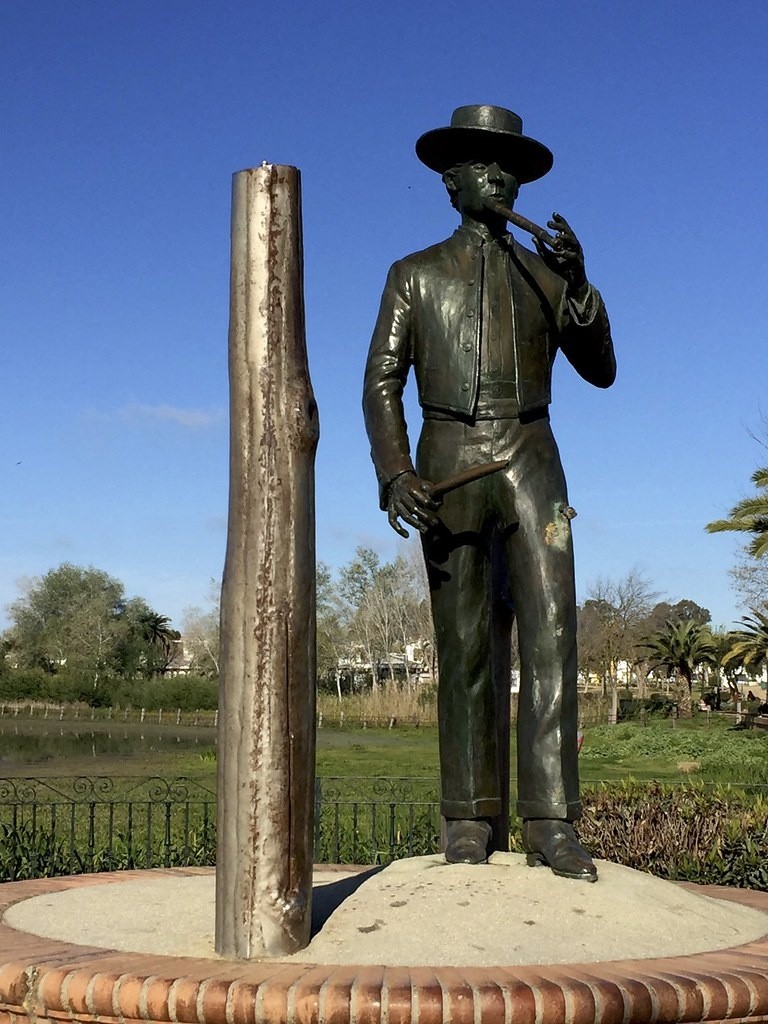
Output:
[362,104,619,882]
